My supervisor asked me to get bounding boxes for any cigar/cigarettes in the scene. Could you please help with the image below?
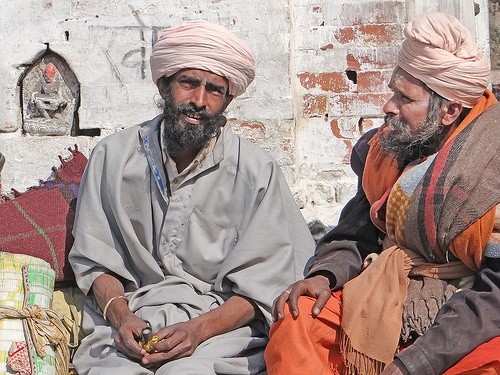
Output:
[141,327,153,345]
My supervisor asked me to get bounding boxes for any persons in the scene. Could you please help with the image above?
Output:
[263,13,500,375]
[30,62,67,123]
[68,21,316,375]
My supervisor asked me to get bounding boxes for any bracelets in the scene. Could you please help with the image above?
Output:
[103,296,129,326]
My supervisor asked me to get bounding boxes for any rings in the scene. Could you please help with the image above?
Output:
[285,289,291,294]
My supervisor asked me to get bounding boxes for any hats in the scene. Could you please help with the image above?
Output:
[397,12,491,108]
[150,21,255,98]
